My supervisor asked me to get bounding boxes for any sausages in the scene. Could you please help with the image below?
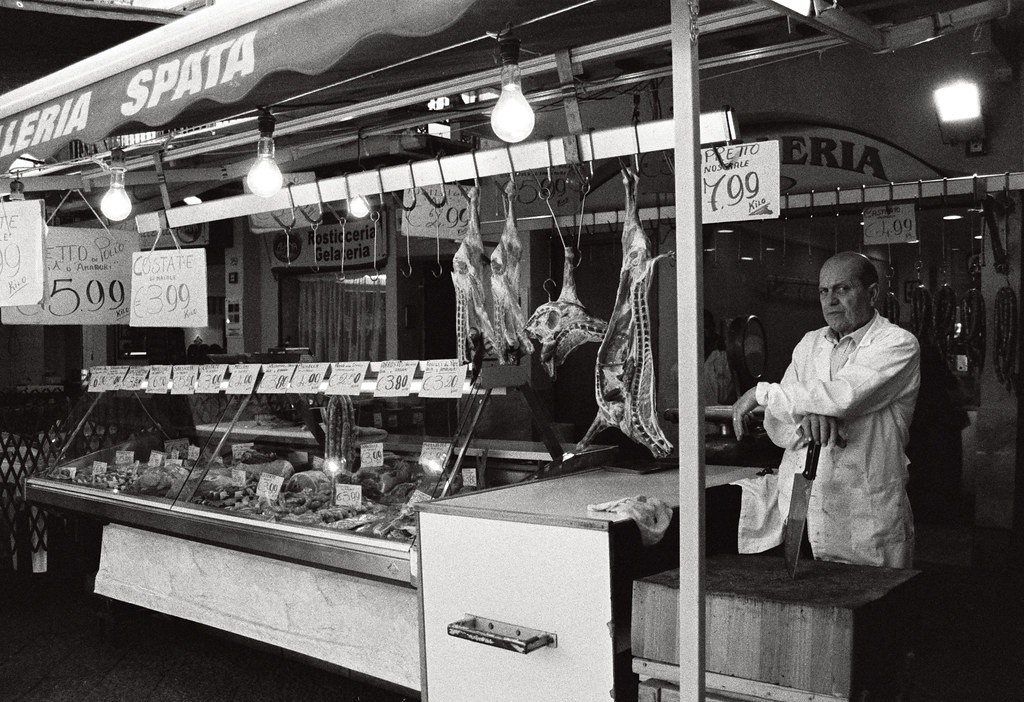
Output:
[246,489,371,526]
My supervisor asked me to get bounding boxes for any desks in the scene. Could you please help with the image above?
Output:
[0,462,47,574]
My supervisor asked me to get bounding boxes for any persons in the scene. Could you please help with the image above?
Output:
[732,252,919,570]
[704,309,742,461]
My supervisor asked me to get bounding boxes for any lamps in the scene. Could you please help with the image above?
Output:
[933,81,986,144]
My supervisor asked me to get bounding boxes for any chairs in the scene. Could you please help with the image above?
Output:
[0,432,25,570]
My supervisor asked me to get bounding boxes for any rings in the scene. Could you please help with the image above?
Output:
[733,418,736,420]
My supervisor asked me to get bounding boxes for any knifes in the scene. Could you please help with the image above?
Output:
[784,437,822,579]
